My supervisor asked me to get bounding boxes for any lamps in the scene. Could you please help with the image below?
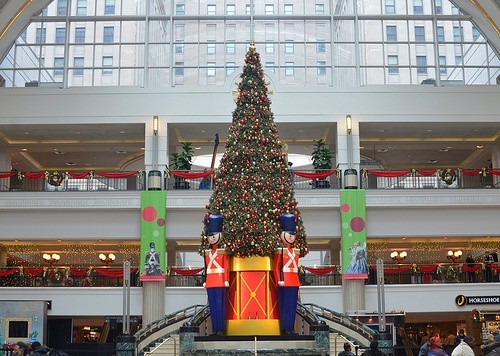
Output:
[346,115,351,134]
[446,250,463,263]
[152,116,158,135]
[390,251,407,264]
[42,253,60,267]
[99,253,115,266]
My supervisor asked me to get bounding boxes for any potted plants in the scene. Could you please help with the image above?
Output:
[310,139,331,189]
[169,142,195,190]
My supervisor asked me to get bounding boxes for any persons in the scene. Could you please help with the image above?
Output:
[338,329,500,356]
[466,254,476,283]
[482,251,494,283]
[0,341,69,356]
